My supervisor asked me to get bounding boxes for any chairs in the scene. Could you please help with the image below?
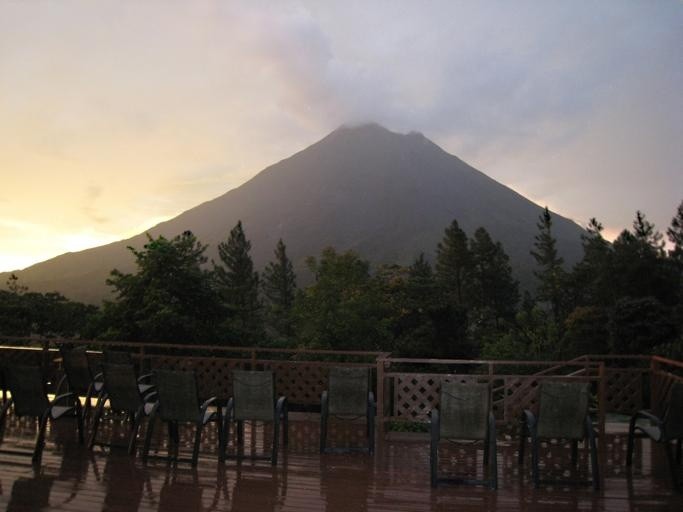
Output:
[84,352,155,457]
[520,375,612,493]
[1,344,85,475]
[315,364,381,455]
[622,375,682,498]
[220,367,289,470]
[142,363,221,472]
[424,378,505,496]
